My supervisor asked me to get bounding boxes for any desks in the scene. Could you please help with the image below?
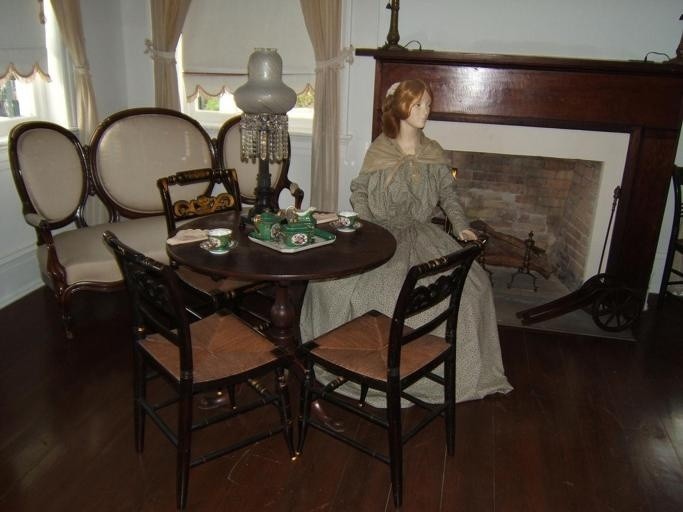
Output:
[167,208,394,431]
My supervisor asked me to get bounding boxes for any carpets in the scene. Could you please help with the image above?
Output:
[471,260,637,339]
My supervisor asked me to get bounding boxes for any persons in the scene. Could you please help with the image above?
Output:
[296,73,515,411]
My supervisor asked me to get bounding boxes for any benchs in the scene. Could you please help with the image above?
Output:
[8,105,302,347]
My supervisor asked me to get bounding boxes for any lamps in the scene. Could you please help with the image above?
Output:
[233,49,296,227]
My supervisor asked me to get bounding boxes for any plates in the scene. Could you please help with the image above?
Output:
[200,241,237,255]
[329,220,362,232]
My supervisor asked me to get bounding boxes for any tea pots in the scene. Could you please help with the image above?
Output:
[250,205,316,246]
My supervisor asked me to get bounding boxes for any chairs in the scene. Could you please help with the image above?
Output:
[298,242,488,508]
[100,230,297,509]
[155,167,275,409]
[656,165,683,311]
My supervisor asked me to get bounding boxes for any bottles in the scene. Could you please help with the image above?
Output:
[2,99,20,117]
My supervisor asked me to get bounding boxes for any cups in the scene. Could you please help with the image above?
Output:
[338,210,358,228]
[208,228,231,250]
